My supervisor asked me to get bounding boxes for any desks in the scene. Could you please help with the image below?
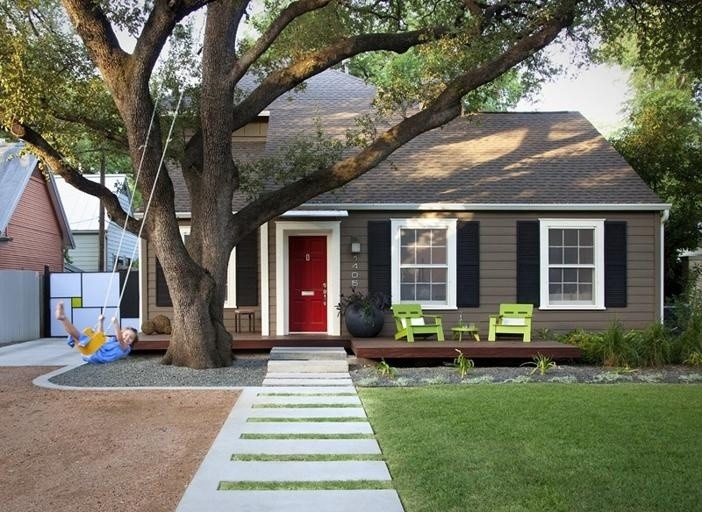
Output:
[449,327,481,342]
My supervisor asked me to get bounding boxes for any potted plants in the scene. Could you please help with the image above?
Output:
[333,286,390,338]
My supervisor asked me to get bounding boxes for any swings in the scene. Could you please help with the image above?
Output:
[75,0,206,355]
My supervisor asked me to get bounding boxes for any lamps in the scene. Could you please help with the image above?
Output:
[351,240,360,254]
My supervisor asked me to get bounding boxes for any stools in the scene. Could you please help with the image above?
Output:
[235,309,256,334]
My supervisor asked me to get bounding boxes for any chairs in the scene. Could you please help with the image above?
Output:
[392,303,445,343]
[488,303,534,342]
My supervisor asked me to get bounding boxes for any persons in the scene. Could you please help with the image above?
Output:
[54,299,139,366]
